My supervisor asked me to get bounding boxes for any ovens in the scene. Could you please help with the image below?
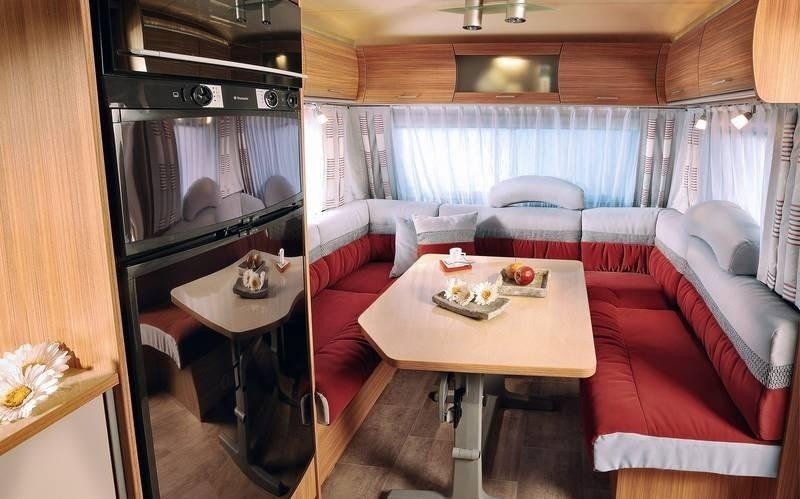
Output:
[87,75,316,499]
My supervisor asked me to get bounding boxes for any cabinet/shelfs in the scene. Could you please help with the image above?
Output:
[662,0,799,103]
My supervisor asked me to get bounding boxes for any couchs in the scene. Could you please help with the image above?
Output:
[308,198,799,499]
[125,193,301,421]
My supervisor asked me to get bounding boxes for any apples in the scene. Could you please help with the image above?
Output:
[246,253,254,264]
[514,266,535,286]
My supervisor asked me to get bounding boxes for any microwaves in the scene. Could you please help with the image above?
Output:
[83,0,315,91]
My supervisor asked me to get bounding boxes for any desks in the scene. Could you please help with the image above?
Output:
[171,249,304,496]
[355,253,597,499]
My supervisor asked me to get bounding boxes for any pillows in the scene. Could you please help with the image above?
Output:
[681,200,762,276]
[183,176,220,222]
[262,175,294,207]
[488,176,585,210]
[389,211,479,278]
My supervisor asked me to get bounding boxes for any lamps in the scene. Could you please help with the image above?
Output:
[439,1,554,31]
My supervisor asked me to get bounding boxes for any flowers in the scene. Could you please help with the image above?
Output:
[0,341,72,426]
[443,276,500,306]
[243,269,266,291]
